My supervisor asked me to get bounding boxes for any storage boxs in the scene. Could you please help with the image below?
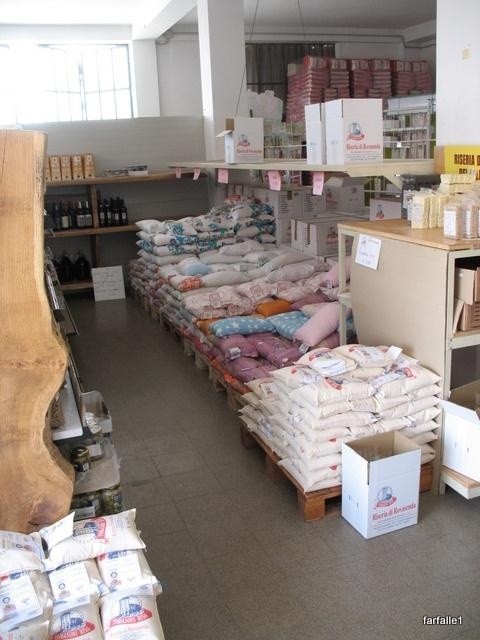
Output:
[456,268,480,331]
[326,98,383,165]
[439,379,480,482]
[61,437,120,495]
[304,102,326,164]
[341,432,422,540]
[216,118,265,165]
[228,177,406,256]
[81,390,113,435]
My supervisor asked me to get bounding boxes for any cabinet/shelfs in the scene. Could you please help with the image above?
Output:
[337,219,480,501]
[45,170,208,292]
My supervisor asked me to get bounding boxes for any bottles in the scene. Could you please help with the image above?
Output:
[51,199,93,231]
[97,197,128,228]
[74,249,89,280]
